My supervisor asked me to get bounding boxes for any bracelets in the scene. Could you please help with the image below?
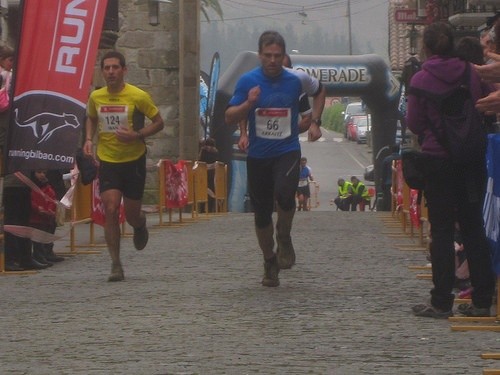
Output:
[136,131,143,139]
[85,138,92,141]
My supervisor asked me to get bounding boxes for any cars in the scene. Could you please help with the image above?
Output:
[347,116,372,143]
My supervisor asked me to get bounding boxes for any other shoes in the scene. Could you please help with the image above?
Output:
[459,303,489,317]
[6,263,24,270]
[25,257,48,269]
[458,287,474,299]
[411,302,452,318]
[34,256,53,266]
[455,259,470,279]
[46,252,64,261]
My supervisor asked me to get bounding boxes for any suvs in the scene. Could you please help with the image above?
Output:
[341,104,368,137]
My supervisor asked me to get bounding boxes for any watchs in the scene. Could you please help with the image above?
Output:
[310,118,322,126]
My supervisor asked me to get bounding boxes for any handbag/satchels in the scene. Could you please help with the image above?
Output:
[401,147,445,189]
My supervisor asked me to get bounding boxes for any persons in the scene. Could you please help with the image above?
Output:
[226,30,303,286]
[332,176,371,212]
[296,156,314,211]
[405,21,494,318]
[83,52,164,281]
[0,42,16,112]
[236,53,327,150]
[454,13,500,134]
[1,167,66,272]
[199,138,220,214]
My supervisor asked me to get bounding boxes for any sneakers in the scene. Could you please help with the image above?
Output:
[107,264,125,280]
[262,262,280,287]
[274,233,296,268]
[133,217,148,249]
